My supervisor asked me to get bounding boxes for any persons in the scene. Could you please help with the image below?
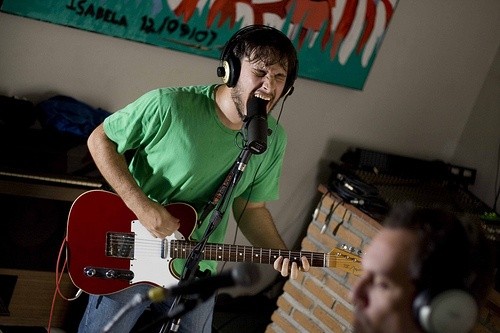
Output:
[76,24,311,333]
[350,207,492,333]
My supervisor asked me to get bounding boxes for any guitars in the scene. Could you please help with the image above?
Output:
[65,189,366,296]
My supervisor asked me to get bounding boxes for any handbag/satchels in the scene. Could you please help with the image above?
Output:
[326,166,391,215]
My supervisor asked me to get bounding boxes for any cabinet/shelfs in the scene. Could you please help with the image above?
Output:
[0,168,102,333]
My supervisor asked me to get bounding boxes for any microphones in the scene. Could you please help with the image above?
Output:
[267,87,294,135]
[245,97,267,154]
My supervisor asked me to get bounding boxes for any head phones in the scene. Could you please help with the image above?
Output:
[414,213,487,333]
[217,24,298,98]
